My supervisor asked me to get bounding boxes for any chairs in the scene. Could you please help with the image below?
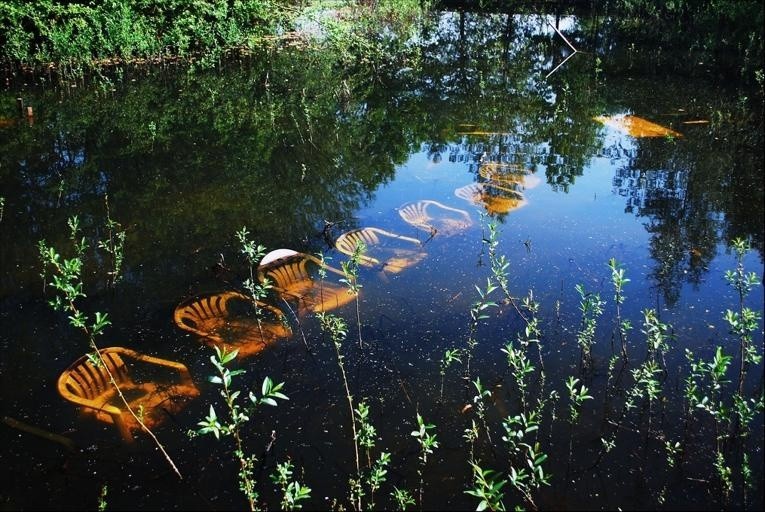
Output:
[335,226,429,285]
[57,344,205,446]
[478,159,540,192]
[454,181,528,227]
[256,246,363,324]
[398,198,475,246]
[169,283,292,370]
